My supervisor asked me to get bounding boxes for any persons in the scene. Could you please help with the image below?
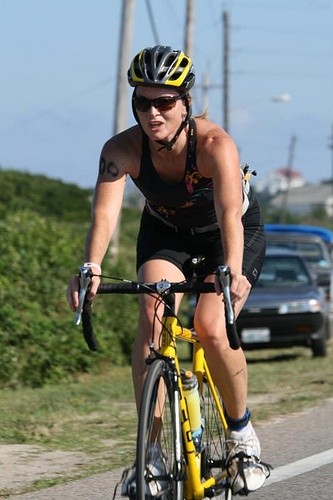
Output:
[66,45,267,497]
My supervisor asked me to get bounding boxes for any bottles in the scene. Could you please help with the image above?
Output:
[191,415,206,450]
[181,371,201,432]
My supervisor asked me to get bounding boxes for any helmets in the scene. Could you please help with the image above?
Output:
[126,45,195,91]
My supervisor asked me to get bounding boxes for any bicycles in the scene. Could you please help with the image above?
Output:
[72,262,274,500]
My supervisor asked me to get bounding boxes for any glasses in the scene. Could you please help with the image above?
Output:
[133,95,182,113]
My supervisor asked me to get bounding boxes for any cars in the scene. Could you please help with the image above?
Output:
[188,249,332,360]
[264,222,333,303]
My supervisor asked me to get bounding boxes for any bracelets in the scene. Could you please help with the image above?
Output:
[84,262,101,271]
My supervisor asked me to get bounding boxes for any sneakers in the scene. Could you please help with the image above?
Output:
[223,421,265,494]
[119,443,168,495]
